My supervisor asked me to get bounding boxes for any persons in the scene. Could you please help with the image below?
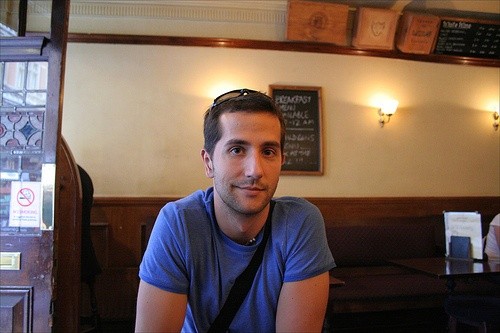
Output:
[134,87,337,333]
[484,213,500,273]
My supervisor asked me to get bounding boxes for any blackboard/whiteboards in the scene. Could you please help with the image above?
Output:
[430,16,500,60]
[268,84,325,176]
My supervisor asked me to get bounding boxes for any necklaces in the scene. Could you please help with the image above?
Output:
[241,218,267,246]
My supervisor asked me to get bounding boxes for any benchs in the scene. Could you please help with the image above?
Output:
[140,213,500,333]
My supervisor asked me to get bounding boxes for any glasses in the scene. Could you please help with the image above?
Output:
[209,88,275,115]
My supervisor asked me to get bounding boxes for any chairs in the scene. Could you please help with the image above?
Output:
[444,296,500,333]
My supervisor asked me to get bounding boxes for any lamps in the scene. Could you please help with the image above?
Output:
[377,99,399,129]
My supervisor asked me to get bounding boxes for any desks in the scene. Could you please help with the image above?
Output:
[386,257,500,333]
